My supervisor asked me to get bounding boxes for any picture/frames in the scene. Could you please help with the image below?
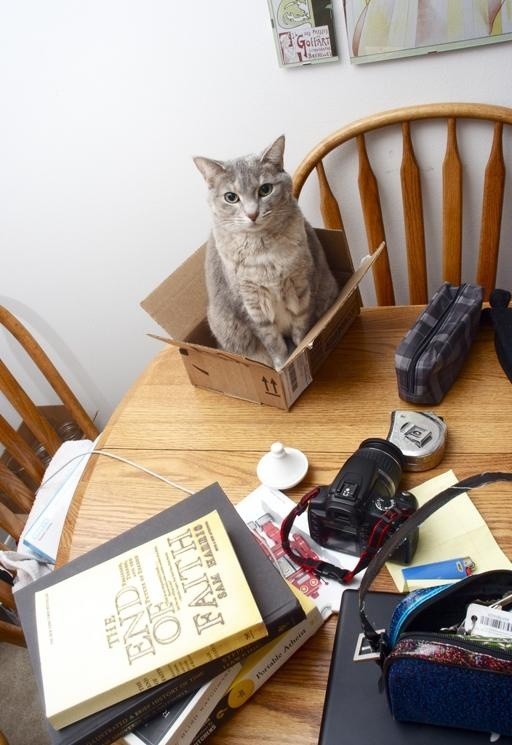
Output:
[268,0,343,67]
[341,0,512,66]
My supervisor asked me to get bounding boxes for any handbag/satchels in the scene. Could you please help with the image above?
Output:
[357,472,511,731]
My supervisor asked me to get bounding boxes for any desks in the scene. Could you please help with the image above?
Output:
[54,306,510,740]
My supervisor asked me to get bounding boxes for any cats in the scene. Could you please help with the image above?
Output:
[192,134,339,371]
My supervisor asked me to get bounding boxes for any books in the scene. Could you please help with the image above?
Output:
[14,482,324,745]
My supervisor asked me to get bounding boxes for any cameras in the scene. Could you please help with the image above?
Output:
[306,436,421,566]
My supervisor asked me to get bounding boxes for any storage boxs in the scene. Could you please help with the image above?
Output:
[6,405,101,517]
[137,227,386,411]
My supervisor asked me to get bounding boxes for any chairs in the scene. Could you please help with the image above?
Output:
[291,102,512,305]
[2,304,98,644]
